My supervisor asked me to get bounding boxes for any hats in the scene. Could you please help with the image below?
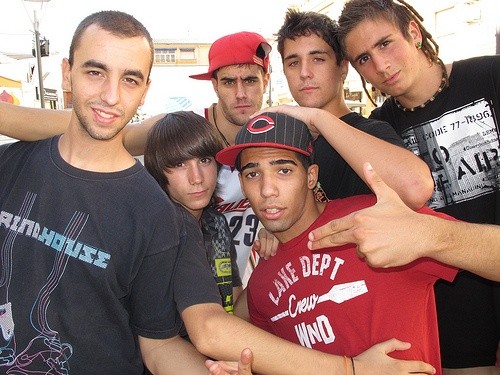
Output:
[189,30,273,80]
[215,110,316,166]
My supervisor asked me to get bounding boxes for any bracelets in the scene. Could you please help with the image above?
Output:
[350,356,356,375]
[344,355,349,375]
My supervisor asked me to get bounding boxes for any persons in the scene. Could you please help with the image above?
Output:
[339,0,500,375]
[0,8,500,375]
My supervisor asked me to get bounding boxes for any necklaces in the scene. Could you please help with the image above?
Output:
[214,102,232,148]
[393,61,447,112]
